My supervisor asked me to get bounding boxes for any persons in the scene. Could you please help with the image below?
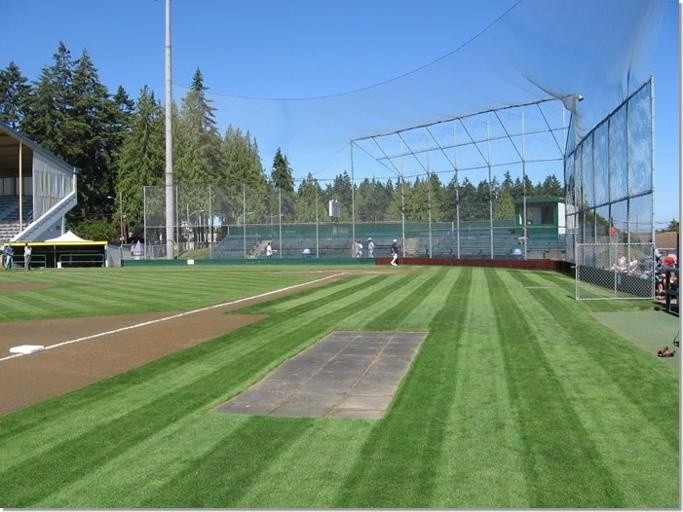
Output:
[0,244,14,270]
[302,248,311,257]
[518,228,529,246]
[266,242,272,259]
[610,238,677,300]
[367,237,374,259]
[390,239,403,268]
[24,242,32,271]
[351,239,363,258]
